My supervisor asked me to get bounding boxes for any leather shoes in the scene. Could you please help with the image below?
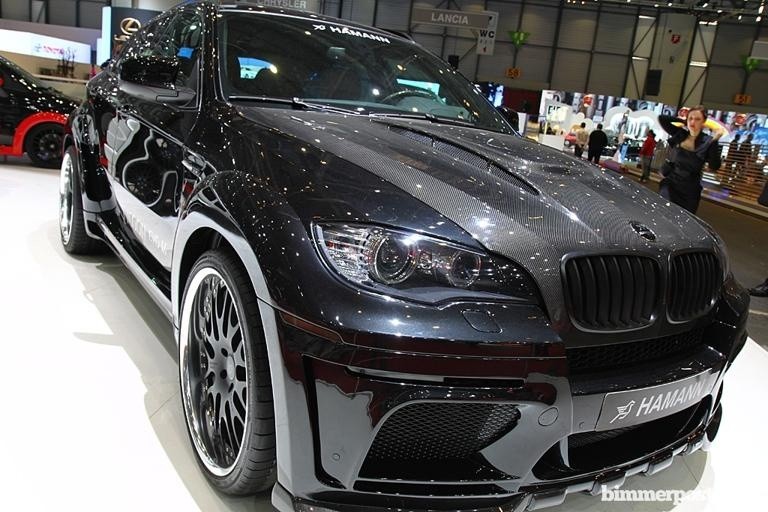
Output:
[747,278,768,299]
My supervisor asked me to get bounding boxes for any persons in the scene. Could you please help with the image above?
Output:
[520,98,768,196]
[658,103,723,215]
